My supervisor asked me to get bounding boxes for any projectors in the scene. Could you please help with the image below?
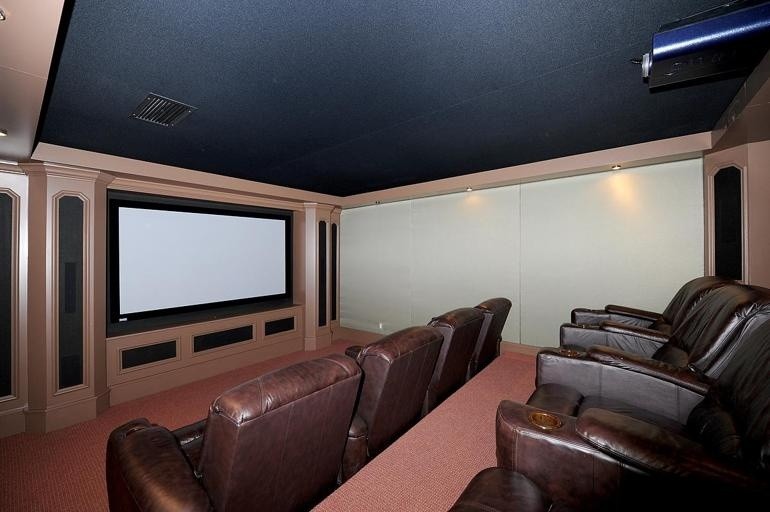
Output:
[640,1,769,93]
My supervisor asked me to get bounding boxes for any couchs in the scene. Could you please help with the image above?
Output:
[107,295,513,512]
[442,274,770,510]
